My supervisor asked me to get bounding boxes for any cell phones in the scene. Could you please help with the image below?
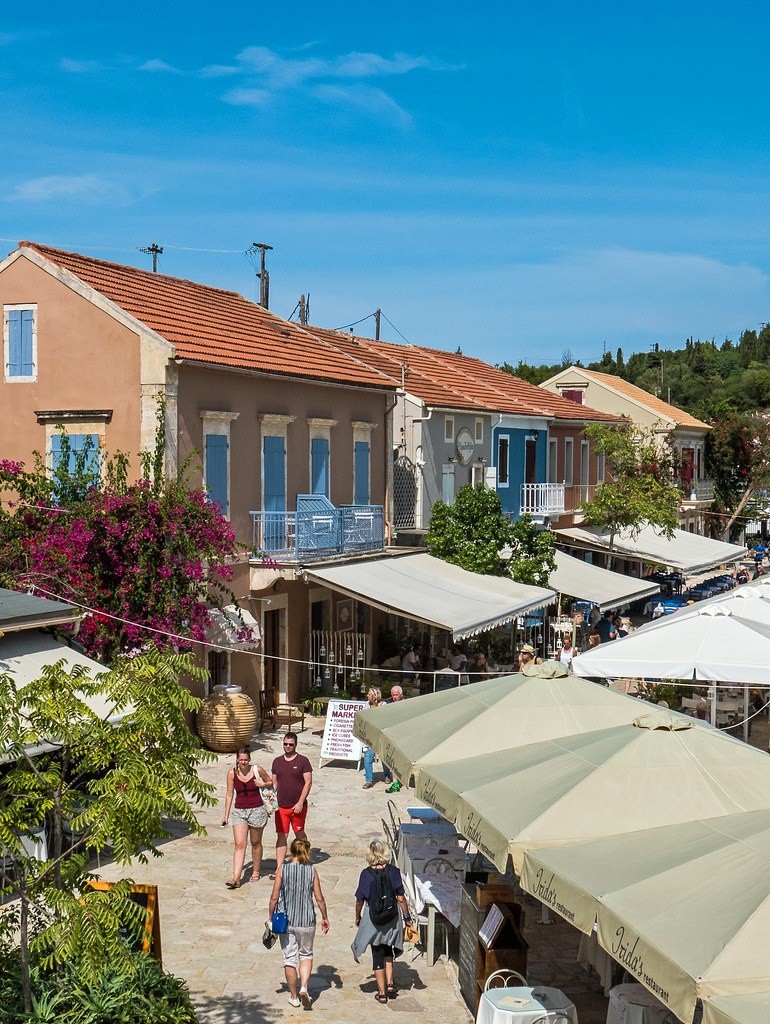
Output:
[220,822,227,828]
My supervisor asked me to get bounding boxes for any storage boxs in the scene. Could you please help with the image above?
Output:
[476,885,514,910]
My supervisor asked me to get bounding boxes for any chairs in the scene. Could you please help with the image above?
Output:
[682,684,762,737]
[630,571,741,615]
[531,1012,573,1024]
[71,829,101,872]
[380,799,520,961]
[258,686,308,736]
[46,807,57,859]
[484,969,528,992]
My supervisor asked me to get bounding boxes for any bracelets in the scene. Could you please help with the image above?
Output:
[404,915,411,921]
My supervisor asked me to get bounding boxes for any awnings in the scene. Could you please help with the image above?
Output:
[307,517,748,642]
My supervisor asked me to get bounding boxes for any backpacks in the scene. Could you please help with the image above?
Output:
[366,863,398,926]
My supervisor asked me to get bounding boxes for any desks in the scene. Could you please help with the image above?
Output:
[3,826,47,868]
[407,806,446,826]
[399,823,459,861]
[414,873,465,967]
[576,930,622,996]
[604,983,667,1023]
[475,986,578,1024]
[404,846,472,888]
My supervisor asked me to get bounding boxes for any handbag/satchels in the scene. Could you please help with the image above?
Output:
[271,911,288,933]
[253,764,279,819]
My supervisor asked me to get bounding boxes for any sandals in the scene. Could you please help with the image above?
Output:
[374,992,388,1004]
[386,984,398,999]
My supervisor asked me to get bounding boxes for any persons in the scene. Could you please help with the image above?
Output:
[268,839,330,1010]
[220,749,274,888]
[384,685,405,794]
[267,732,313,881]
[355,840,412,1004]
[363,688,387,789]
[373,531,770,709]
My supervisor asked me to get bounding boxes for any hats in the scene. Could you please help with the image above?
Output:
[519,643,537,656]
[403,924,420,944]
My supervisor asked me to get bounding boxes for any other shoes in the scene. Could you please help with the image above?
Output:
[298,988,314,1010]
[383,777,391,784]
[385,780,402,793]
[269,871,277,880]
[362,781,372,789]
[287,994,301,1007]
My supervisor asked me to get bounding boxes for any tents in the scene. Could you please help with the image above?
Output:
[569,573,770,742]
[356,671,770,1024]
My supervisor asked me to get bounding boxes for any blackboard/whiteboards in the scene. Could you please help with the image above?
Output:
[433,667,460,693]
[83,880,162,968]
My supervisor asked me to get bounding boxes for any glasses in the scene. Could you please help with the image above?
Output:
[283,742,294,746]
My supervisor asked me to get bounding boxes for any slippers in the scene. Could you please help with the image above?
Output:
[247,874,263,883]
[225,880,241,888]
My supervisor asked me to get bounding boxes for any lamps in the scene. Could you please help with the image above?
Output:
[273,580,281,592]
